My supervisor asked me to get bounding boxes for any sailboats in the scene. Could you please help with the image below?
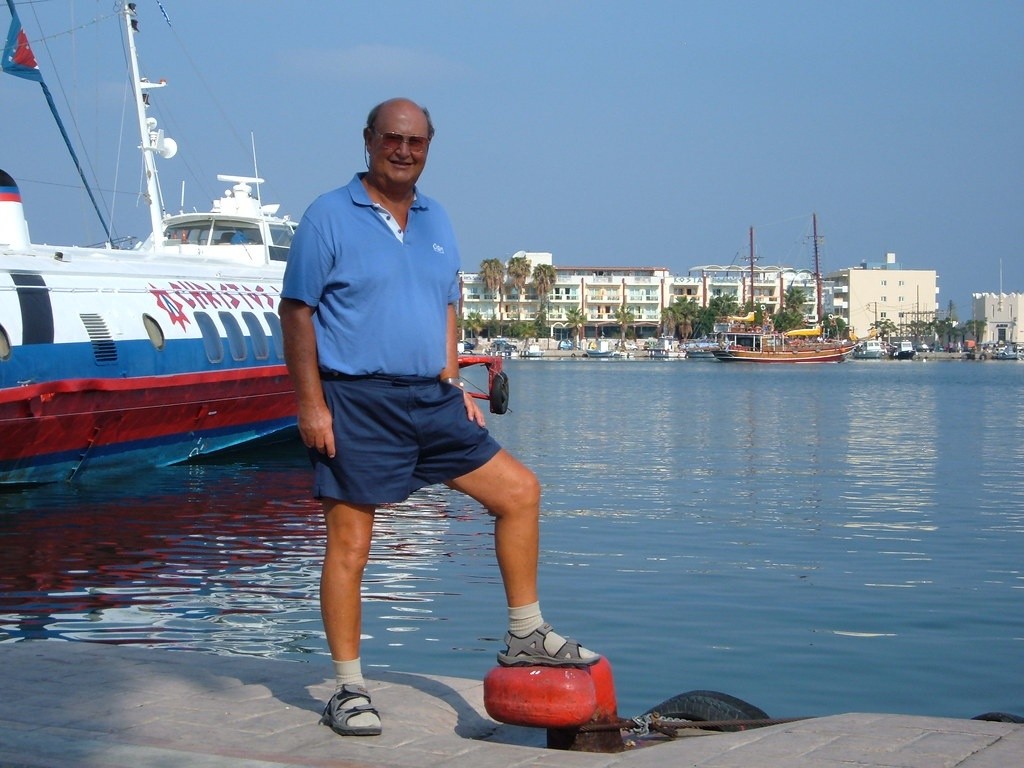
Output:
[644,212,863,364]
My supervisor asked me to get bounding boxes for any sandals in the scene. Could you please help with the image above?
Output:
[323,684,382,735]
[497,623,601,669]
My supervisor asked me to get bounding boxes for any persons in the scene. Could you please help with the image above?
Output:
[787,334,825,346]
[947,341,998,352]
[279,98,602,737]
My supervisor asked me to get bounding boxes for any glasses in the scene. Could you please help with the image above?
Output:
[372,128,431,153]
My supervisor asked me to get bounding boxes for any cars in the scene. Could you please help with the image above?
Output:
[558,339,582,351]
[458,339,475,351]
[489,339,518,351]
[879,340,898,353]
[914,341,964,354]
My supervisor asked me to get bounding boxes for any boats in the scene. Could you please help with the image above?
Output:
[853,340,885,359]
[519,349,547,358]
[889,340,916,360]
[583,347,617,358]
[958,344,1024,361]
[1,3,511,496]
[481,347,512,358]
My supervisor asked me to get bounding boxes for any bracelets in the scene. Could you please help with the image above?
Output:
[441,377,463,386]
